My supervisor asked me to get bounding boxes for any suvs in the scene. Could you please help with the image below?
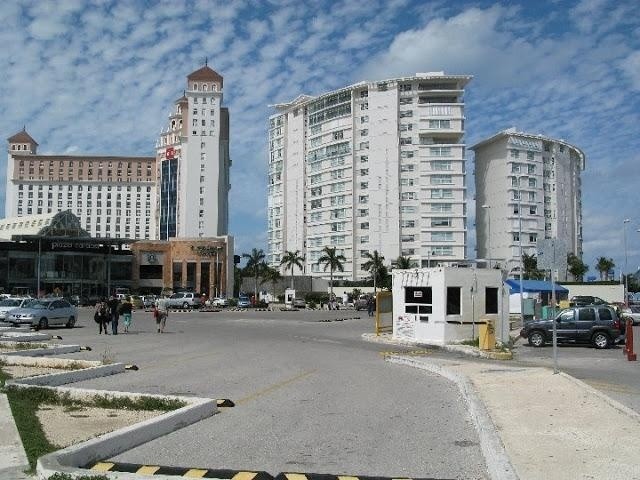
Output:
[354,293,377,310]
[520,291,640,347]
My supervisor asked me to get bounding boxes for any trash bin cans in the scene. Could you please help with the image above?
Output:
[478,319,496,350]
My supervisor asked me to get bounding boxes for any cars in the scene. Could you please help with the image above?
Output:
[112,287,253,310]
[0,294,88,330]
[291,298,306,309]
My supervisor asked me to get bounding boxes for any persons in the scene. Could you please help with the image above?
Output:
[154,294,170,333]
[121,297,133,334]
[94,292,111,335]
[200,292,207,308]
[341,290,349,306]
[106,293,122,335]
[367,296,375,317]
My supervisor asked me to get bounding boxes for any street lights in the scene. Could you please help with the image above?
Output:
[215,246,222,297]
[480,204,492,268]
[624,218,630,310]
[427,250,436,268]
[517,171,529,332]
